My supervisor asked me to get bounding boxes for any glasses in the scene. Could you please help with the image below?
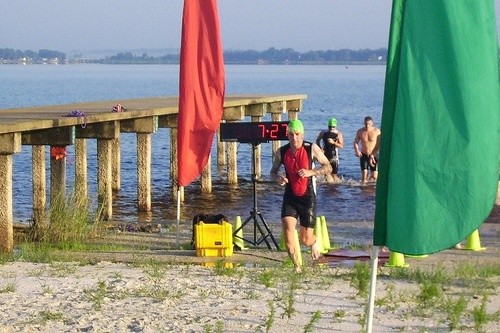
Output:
[329,127,336,129]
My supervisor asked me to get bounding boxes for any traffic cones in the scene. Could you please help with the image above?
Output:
[319,215,336,250]
[304,217,329,254]
[383,251,409,268]
[231,216,250,251]
[458,229,486,251]
[274,227,287,252]
[281,230,304,267]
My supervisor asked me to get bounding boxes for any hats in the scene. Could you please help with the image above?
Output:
[328,118,337,127]
[288,118,304,133]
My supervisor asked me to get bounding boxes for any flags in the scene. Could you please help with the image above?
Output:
[373,0,500,256]
[177,0,224,186]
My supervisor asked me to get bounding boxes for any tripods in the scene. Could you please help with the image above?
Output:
[232,144,280,252]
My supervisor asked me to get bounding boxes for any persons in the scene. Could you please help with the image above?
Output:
[273,120,333,274]
[316,117,343,183]
[369,135,381,168]
[354,116,381,182]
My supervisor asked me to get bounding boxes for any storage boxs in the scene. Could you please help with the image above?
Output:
[194,220,234,258]
